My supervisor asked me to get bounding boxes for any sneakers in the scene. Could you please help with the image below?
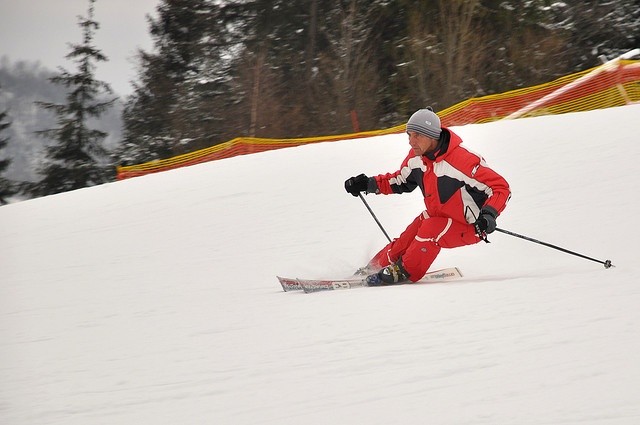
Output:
[378,256,410,284]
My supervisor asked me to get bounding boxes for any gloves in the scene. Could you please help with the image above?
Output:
[479,205,498,233]
[345,174,377,197]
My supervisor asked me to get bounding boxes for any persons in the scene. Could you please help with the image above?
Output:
[344,106,513,286]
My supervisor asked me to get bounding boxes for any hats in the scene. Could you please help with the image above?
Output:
[406,106,442,140]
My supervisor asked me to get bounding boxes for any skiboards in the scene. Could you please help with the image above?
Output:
[276,266,464,294]
